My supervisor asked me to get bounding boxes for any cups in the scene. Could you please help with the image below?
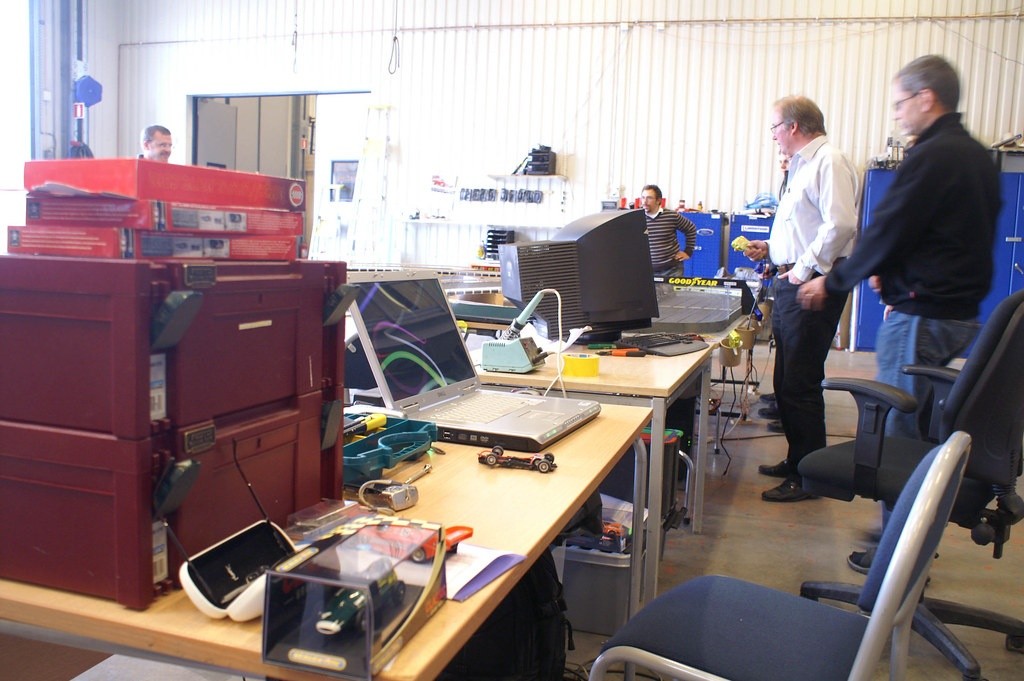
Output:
[736,326,756,350]
[749,298,773,332]
[719,339,743,366]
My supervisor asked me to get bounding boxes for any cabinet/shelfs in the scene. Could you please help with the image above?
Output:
[849,166,1024,352]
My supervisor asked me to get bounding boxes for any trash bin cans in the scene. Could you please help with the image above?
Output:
[598,428,683,561]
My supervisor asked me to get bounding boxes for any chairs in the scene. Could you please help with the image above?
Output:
[589,431,972,681]
[798,289,1024,681]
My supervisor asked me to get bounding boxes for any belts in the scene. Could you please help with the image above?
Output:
[775,257,846,275]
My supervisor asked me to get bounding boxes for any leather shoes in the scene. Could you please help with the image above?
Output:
[760,392,777,402]
[767,419,785,433]
[761,478,819,502]
[758,407,782,420]
[757,458,793,478]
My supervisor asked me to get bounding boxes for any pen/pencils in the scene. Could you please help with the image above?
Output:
[596,348,646,357]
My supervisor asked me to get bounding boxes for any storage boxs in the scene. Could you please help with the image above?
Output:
[989,149,1024,172]
[0,158,347,609]
[553,493,648,636]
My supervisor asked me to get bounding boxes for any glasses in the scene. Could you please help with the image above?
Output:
[889,90,920,110]
[152,438,290,606]
[768,120,785,134]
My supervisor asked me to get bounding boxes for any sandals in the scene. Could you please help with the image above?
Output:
[845,549,932,583]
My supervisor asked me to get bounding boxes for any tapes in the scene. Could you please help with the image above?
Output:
[560,353,599,378]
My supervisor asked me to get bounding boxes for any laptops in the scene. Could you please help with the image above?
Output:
[346,270,601,453]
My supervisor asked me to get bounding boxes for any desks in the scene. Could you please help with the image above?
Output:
[0,310,760,681]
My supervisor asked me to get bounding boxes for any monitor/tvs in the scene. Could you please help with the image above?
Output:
[497,206,659,343]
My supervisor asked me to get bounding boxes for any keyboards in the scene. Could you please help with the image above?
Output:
[615,332,709,357]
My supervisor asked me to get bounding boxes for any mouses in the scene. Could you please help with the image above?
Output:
[684,333,705,343]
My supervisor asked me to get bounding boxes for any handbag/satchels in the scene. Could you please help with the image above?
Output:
[437,546,575,681]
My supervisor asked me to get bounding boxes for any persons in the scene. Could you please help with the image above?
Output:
[640,185,697,275]
[138,125,173,163]
[743,94,860,502]
[795,54,1002,575]
[779,154,792,201]
